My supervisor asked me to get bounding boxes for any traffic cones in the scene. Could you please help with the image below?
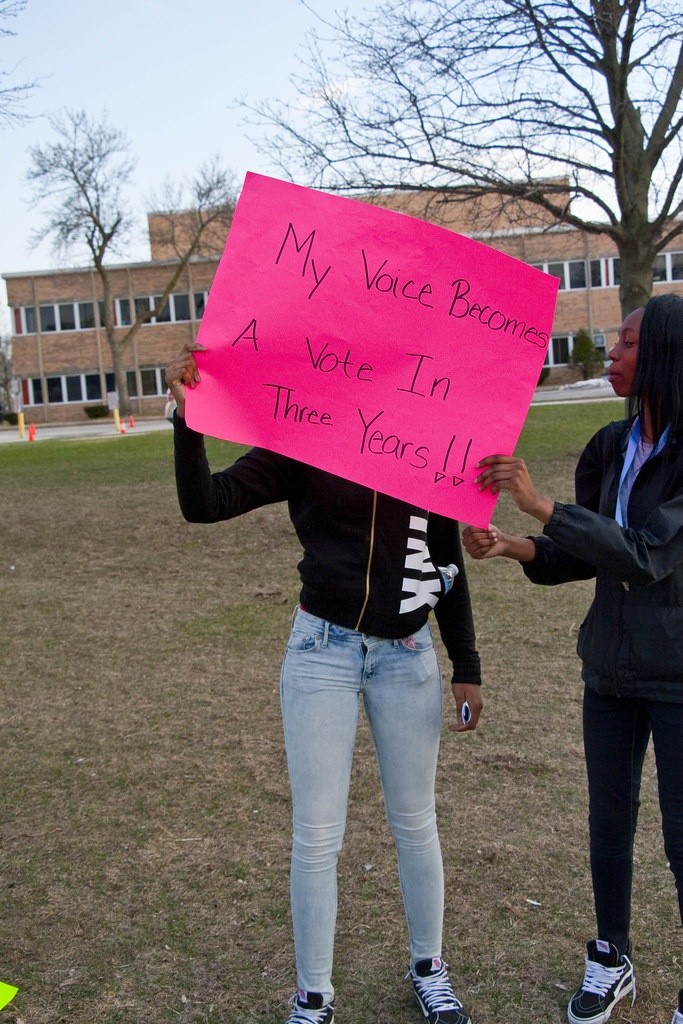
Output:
[129,415,135,429]
[120,418,128,436]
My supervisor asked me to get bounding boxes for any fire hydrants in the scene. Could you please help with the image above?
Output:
[28,422,37,442]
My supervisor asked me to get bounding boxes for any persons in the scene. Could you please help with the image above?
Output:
[461,294,683,1023]
[164,342,485,1024]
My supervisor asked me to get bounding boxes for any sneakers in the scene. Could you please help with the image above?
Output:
[283,991,337,1024]
[567,940,637,1024]
[402,952,471,1024]
